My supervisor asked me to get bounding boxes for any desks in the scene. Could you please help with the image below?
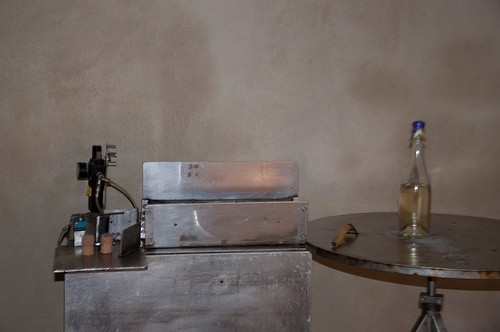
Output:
[305,210,500,332]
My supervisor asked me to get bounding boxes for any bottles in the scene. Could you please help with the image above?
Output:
[399,121,431,238]
